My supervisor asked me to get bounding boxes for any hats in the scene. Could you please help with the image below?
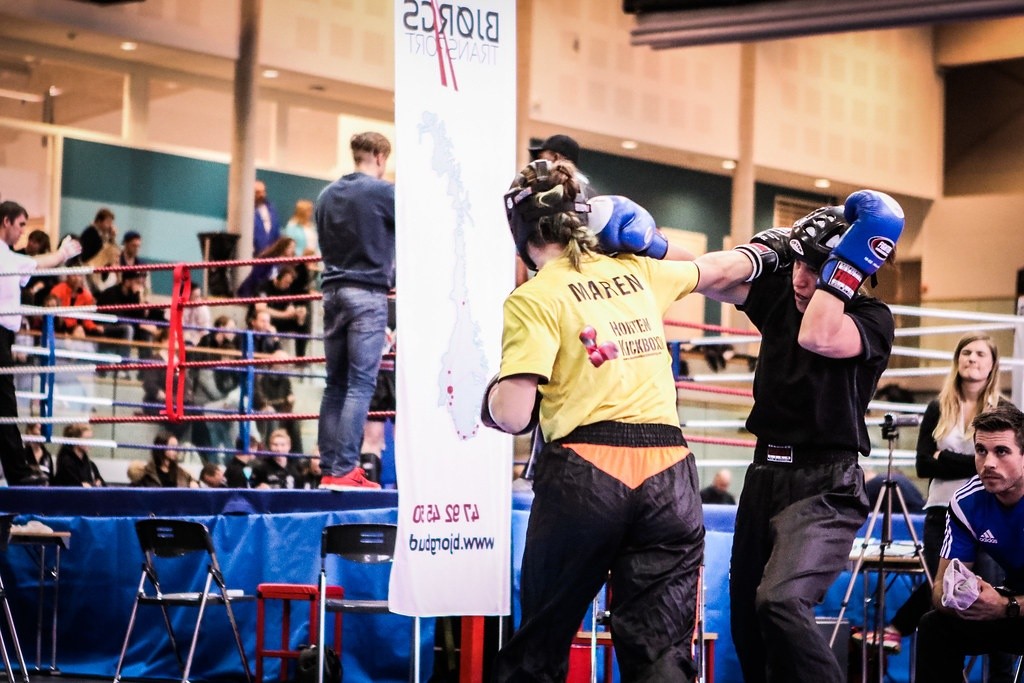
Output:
[527,135,580,164]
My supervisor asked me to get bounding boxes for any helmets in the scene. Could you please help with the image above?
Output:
[787,201,852,266]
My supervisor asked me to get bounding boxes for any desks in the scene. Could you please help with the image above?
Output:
[8,532,71,677]
[845,556,927,683]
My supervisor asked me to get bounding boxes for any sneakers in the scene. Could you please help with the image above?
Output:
[318,467,382,490]
[850,625,902,654]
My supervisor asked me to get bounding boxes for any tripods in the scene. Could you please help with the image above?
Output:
[828,425,967,683]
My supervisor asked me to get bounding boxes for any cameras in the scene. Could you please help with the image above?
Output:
[883,414,919,427]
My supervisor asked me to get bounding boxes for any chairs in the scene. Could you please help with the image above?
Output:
[112,519,253,683]
[317,523,422,683]
[589,559,718,683]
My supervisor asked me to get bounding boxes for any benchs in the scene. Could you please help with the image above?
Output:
[7,304,206,480]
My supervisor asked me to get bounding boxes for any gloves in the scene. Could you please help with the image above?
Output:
[732,227,794,283]
[586,195,669,260]
[816,189,905,304]
[58,234,82,264]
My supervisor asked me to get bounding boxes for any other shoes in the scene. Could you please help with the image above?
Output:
[11,473,49,486]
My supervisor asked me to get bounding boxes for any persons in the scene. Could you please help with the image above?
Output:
[700,468,736,505]
[0,200,247,491]
[587,189,905,683]
[849,331,1024,683]
[360,284,395,483]
[480,155,787,683]
[226,180,323,490]
[312,130,395,490]
[516,134,580,289]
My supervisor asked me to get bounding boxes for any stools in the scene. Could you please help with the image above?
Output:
[256,583,343,682]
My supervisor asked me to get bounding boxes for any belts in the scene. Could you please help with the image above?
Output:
[751,445,834,468]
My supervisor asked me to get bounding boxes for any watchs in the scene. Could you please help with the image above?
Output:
[1004,594,1020,620]
